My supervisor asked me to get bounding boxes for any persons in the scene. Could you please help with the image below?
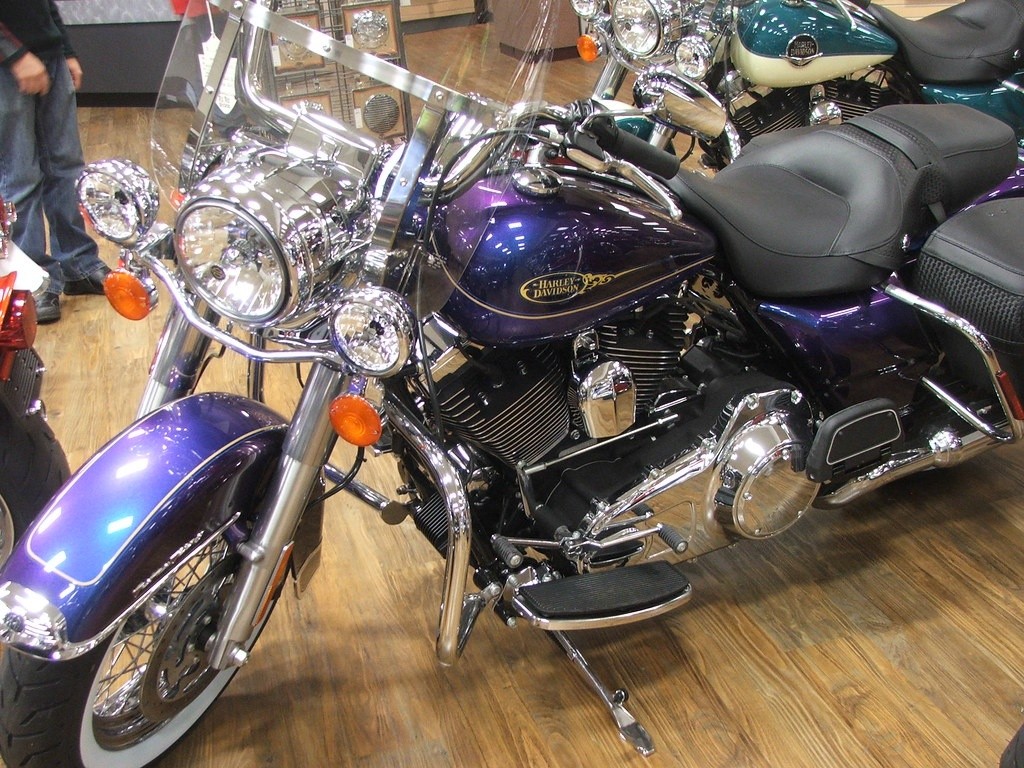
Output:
[171,0,288,142]
[0,0,112,325]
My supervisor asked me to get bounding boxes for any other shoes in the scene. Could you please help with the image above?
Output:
[62,267,112,295]
[35,292,61,322]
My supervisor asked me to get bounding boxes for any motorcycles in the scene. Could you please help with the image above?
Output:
[0,0,1024,768]
